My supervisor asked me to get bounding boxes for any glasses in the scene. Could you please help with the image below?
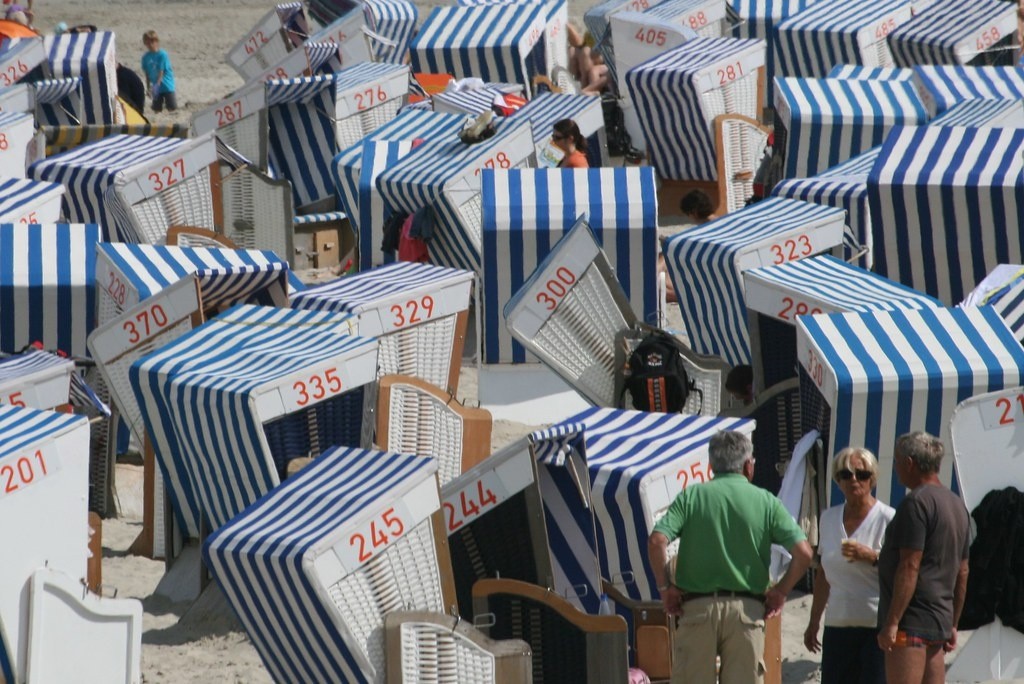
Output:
[552,134,570,142]
[838,469,873,481]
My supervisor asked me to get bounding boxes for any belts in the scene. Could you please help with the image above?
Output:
[682,590,766,603]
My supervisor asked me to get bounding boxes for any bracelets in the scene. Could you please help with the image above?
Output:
[871,550,879,567]
[656,580,671,591]
[776,584,788,596]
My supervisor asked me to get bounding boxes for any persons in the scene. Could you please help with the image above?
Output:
[552,118,596,168]
[648,430,814,684]
[877,430,969,684]
[141,29,177,112]
[566,22,609,93]
[659,189,718,303]
[804,447,897,684]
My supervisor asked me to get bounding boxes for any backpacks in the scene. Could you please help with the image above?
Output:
[628,329,690,412]
